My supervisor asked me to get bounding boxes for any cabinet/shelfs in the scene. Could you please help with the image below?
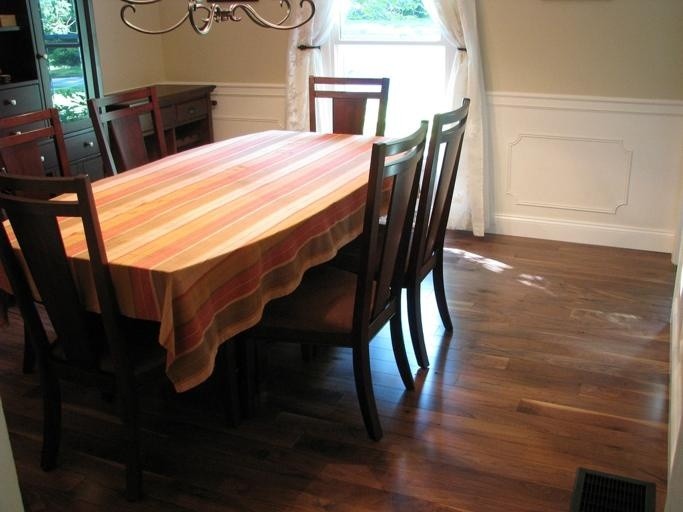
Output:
[0,0,111,223]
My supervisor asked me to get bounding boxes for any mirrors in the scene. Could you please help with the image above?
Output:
[38,0,96,125]
